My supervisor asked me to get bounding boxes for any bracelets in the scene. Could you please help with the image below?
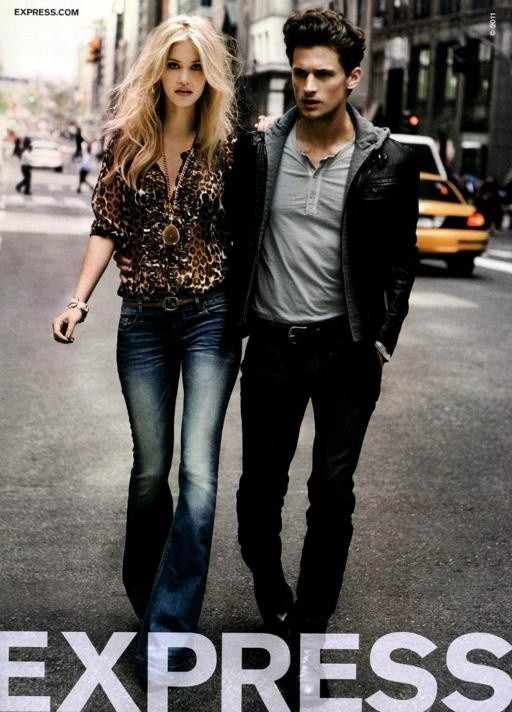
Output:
[66,297,89,323]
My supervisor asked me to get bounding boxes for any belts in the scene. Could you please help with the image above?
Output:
[122,289,223,312]
[255,316,348,345]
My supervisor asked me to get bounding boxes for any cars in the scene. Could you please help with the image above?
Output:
[387,134,489,275]
[30,140,64,172]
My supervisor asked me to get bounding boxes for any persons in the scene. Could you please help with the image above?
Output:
[53,14,249,692]
[13,137,33,194]
[71,128,94,193]
[219,8,420,706]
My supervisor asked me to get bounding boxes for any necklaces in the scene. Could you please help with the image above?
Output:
[162,148,195,246]
